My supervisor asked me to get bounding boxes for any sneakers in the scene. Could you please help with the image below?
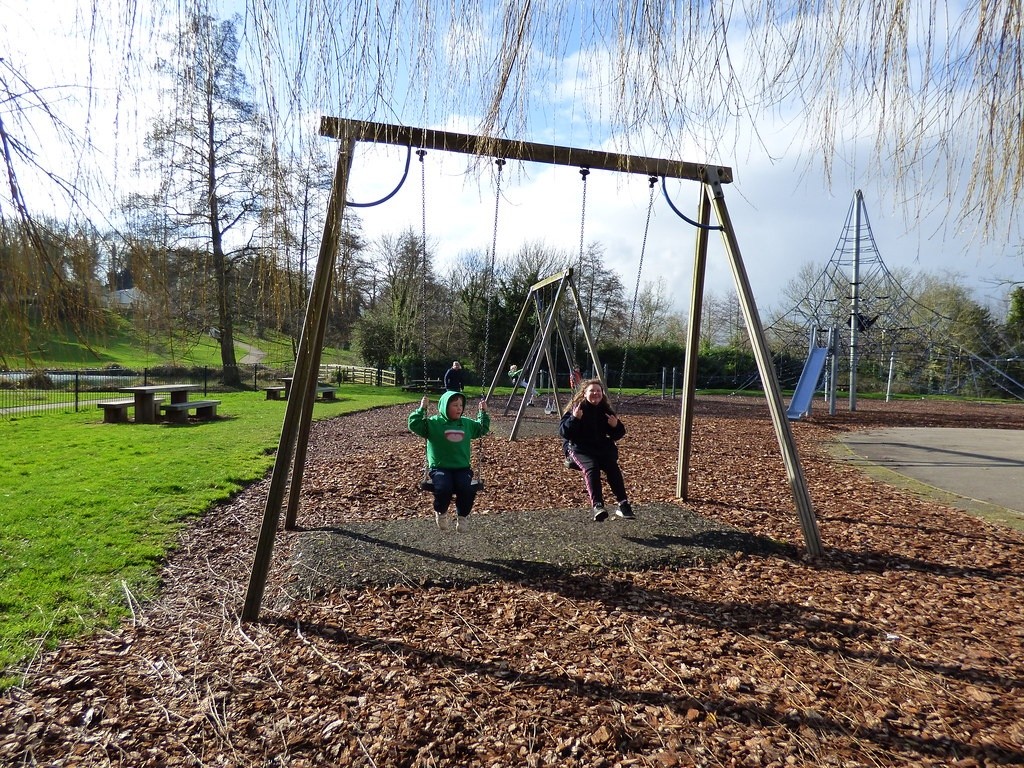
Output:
[456,514,469,533]
[615,501,633,518]
[593,504,608,523]
[434,510,448,530]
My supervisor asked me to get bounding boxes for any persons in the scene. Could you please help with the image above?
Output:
[558,380,636,522]
[570,365,581,391]
[508,365,541,398]
[408,391,490,533]
[444,361,465,392]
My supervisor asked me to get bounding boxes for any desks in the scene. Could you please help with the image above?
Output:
[408,380,442,392]
[277,377,327,398]
[118,384,201,422]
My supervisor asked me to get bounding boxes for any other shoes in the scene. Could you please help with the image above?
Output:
[535,393,540,398]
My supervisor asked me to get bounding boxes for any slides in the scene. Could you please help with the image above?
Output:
[784,348,828,421]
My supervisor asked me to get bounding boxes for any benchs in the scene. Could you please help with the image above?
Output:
[97,398,164,423]
[164,400,222,423]
[402,385,446,393]
[316,387,339,400]
[264,387,286,399]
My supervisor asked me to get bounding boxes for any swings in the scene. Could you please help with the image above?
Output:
[414,149,507,493]
[512,313,550,387]
[562,168,660,471]
